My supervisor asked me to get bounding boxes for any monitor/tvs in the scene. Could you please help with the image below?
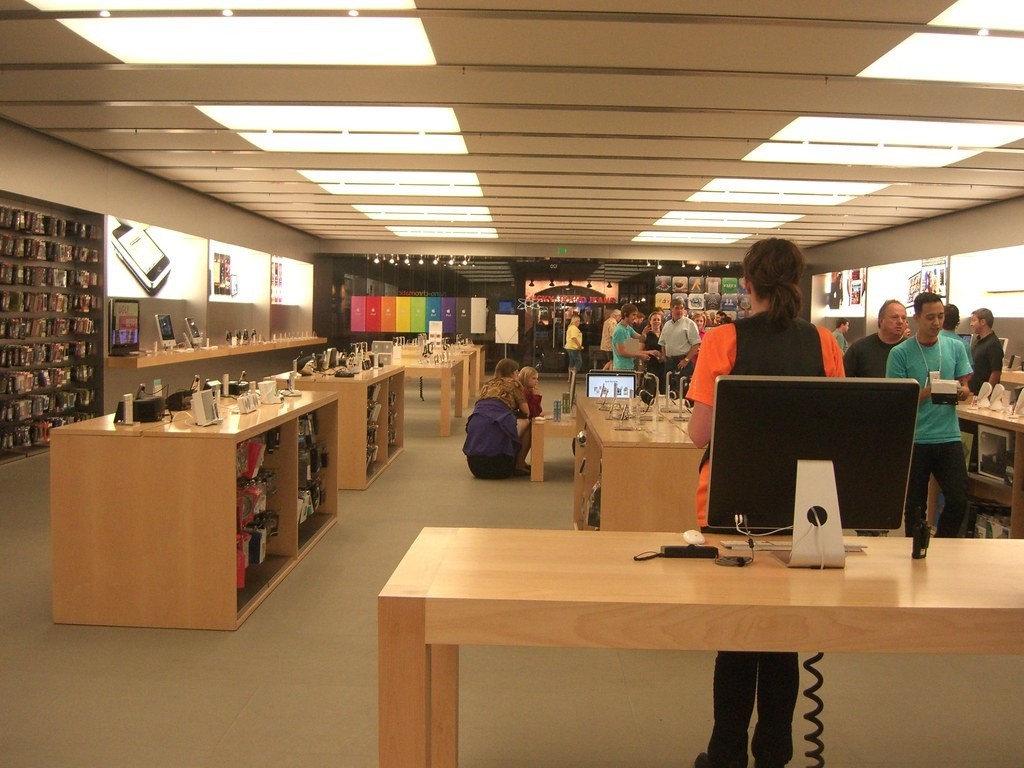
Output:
[999,338,1008,356]
[957,333,973,348]
[586,372,637,399]
[706,375,920,567]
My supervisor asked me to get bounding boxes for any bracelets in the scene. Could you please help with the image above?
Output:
[685,358,690,362]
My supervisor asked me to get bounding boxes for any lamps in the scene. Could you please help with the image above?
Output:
[645,260,731,271]
[566,281,574,289]
[606,281,613,288]
[587,281,593,289]
[373,253,476,268]
[549,280,555,287]
[528,280,535,287]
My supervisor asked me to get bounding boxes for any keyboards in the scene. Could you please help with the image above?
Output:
[719,541,869,552]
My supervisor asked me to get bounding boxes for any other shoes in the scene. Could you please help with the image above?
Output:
[695,752,713,768]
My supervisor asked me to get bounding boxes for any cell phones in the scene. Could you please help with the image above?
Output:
[184,317,202,344]
[111,225,172,295]
[849,280,862,306]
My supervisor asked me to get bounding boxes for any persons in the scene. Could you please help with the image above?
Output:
[832,318,849,354]
[563,314,584,384]
[842,299,909,536]
[904,319,911,338]
[692,311,731,339]
[461,358,543,477]
[938,303,973,367]
[884,292,974,538]
[600,298,702,407]
[685,235,846,768]
[968,308,1004,399]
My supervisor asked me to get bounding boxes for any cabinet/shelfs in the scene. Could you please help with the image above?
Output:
[0,201,104,462]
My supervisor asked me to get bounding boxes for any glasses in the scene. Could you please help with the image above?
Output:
[673,306,684,311]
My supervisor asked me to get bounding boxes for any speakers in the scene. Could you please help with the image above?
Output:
[191,390,217,426]
[976,382,1005,409]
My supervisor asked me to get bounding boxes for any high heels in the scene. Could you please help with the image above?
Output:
[514,462,531,477]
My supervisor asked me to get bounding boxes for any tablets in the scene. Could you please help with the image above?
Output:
[154,314,176,348]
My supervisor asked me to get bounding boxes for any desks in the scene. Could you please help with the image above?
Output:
[376,527,1024,768]
[47,386,341,631]
[530,412,577,481]
[391,341,485,436]
[574,397,709,532]
[955,403,1024,539]
[106,335,327,368]
[262,363,405,490]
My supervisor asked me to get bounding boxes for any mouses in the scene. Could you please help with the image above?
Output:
[683,530,705,545]
